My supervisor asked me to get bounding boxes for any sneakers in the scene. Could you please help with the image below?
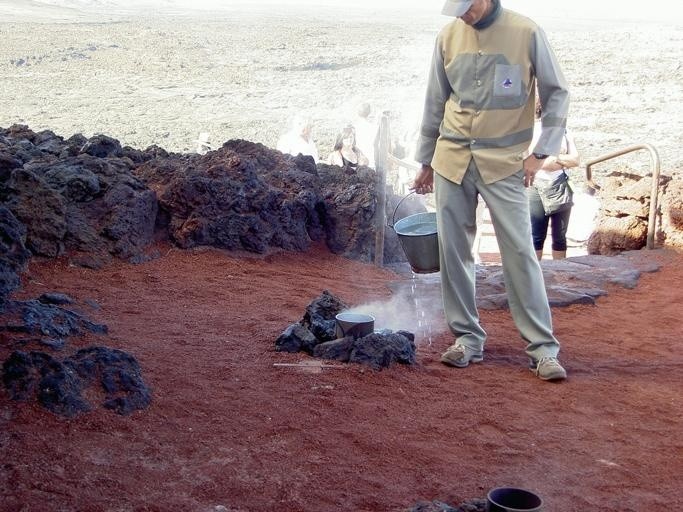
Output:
[528,357,568,382]
[439,341,484,368]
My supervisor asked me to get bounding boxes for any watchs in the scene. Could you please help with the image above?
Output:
[533,152,546,159]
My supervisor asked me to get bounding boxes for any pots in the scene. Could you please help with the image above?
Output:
[332,312,375,340]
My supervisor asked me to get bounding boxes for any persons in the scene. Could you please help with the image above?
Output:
[195,100,415,193]
[409,0,569,383]
[527,98,580,260]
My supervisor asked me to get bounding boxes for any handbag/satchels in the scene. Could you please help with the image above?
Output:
[531,166,575,217]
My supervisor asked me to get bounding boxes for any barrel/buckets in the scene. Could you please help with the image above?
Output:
[388,190,441,273]
[335,311,375,338]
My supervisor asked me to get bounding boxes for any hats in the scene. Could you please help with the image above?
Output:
[439,0,476,17]
[190,132,218,149]
